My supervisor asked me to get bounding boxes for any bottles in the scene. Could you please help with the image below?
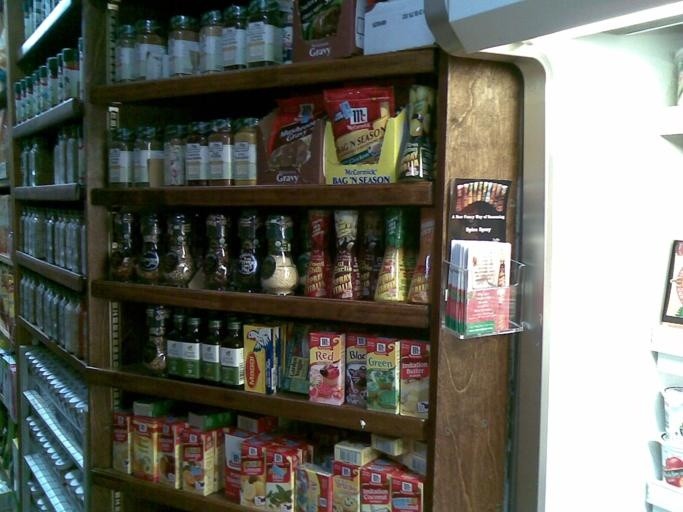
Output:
[6,232,13,256]
[496,259,505,306]
[18,272,85,357]
[106,119,262,188]
[112,0,292,85]
[164,305,243,389]
[13,126,87,186]
[18,208,85,274]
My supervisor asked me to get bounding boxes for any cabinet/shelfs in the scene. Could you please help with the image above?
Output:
[91,43,520,512]
[1,0,88,512]
[643,103,683,512]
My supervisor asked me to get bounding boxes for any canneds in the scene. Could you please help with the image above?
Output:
[107,117,259,186]
[25,350,88,512]
[114,1,282,84]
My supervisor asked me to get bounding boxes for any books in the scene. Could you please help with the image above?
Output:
[444,239,511,334]
